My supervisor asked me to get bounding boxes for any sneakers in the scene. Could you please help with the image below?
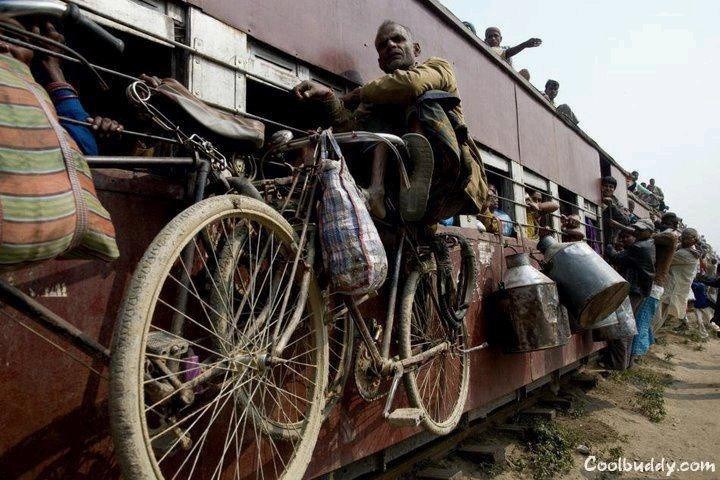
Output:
[398,131,435,222]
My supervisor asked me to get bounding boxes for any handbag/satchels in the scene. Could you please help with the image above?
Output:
[0,53,121,266]
[314,126,389,298]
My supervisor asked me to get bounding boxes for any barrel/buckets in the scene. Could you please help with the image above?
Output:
[529,229,630,331]
[493,245,569,355]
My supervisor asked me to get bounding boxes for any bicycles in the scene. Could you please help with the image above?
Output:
[109,131,488,479]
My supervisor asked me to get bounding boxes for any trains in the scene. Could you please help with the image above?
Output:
[0,0,698,479]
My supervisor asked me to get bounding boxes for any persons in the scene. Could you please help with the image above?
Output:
[1,1,720,365]
